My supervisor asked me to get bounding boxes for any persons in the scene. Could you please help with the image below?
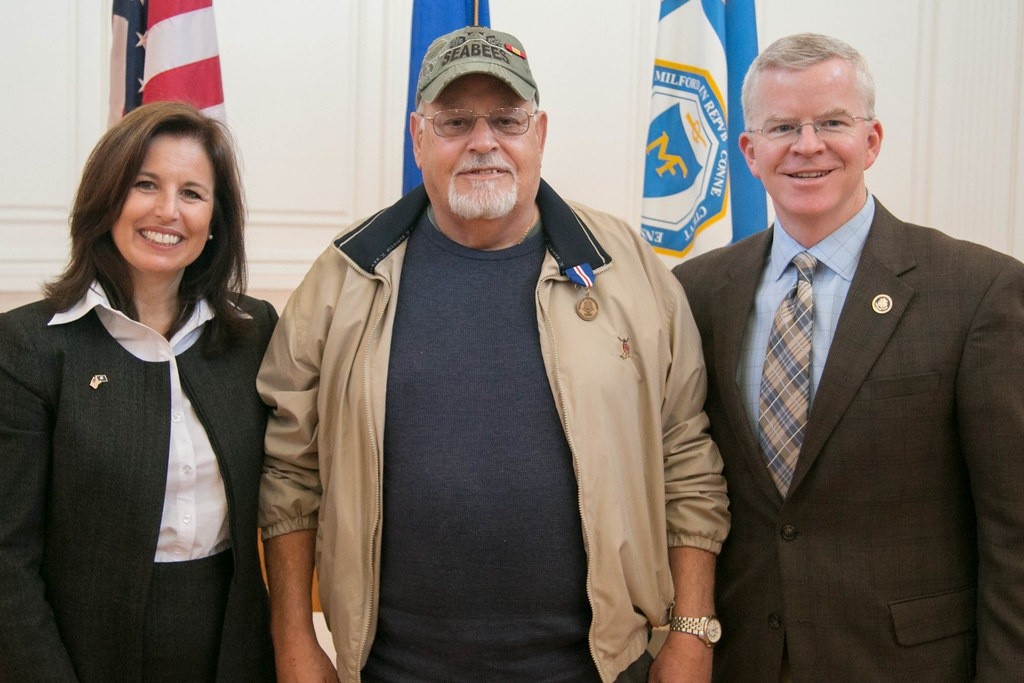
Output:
[1,100,279,683]
[668,33,1024,683]
[255,25,729,682]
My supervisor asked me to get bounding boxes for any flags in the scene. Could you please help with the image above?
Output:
[402,0,491,198]
[639,0,768,271]
[107,0,227,136]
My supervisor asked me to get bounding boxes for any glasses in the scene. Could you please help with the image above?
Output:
[422,108,535,138]
[750,114,869,144]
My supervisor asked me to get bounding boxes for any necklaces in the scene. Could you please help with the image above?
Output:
[518,223,535,244]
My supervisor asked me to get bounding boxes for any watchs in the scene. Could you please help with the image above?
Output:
[670,613,722,649]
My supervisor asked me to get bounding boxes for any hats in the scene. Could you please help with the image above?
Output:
[415,27,539,106]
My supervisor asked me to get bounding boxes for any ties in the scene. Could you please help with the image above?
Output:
[757,252,819,499]
